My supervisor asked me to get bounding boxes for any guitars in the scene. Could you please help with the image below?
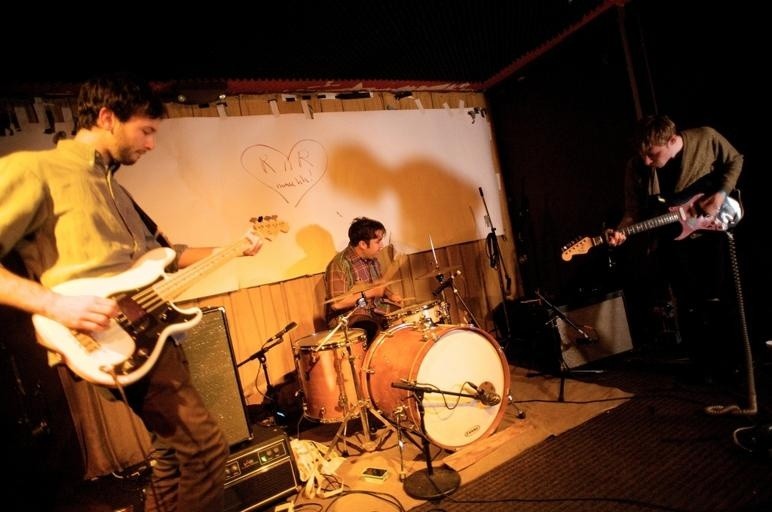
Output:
[32,216,289,388]
[560,173,744,261]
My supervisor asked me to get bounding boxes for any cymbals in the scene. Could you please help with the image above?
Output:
[415,265,462,281]
[321,279,402,305]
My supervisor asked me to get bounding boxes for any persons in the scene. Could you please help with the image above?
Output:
[324,216,406,346]
[602,113,746,384]
[1,68,274,512]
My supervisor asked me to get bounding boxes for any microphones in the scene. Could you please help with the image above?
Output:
[432,270,461,296]
[468,381,500,406]
[505,279,511,297]
[267,322,296,342]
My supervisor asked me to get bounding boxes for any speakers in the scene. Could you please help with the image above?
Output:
[175,305,251,449]
[545,290,638,372]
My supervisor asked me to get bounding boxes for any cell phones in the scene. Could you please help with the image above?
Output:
[360,466,389,479]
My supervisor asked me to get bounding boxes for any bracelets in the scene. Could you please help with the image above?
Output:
[361,290,366,302]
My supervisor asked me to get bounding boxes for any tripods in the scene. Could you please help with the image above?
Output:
[313,291,422,460]
[526,291,607,402]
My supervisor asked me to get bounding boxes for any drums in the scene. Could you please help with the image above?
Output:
[361,321,511,451]
[295,328,368,424]
[386,300,447,329]
